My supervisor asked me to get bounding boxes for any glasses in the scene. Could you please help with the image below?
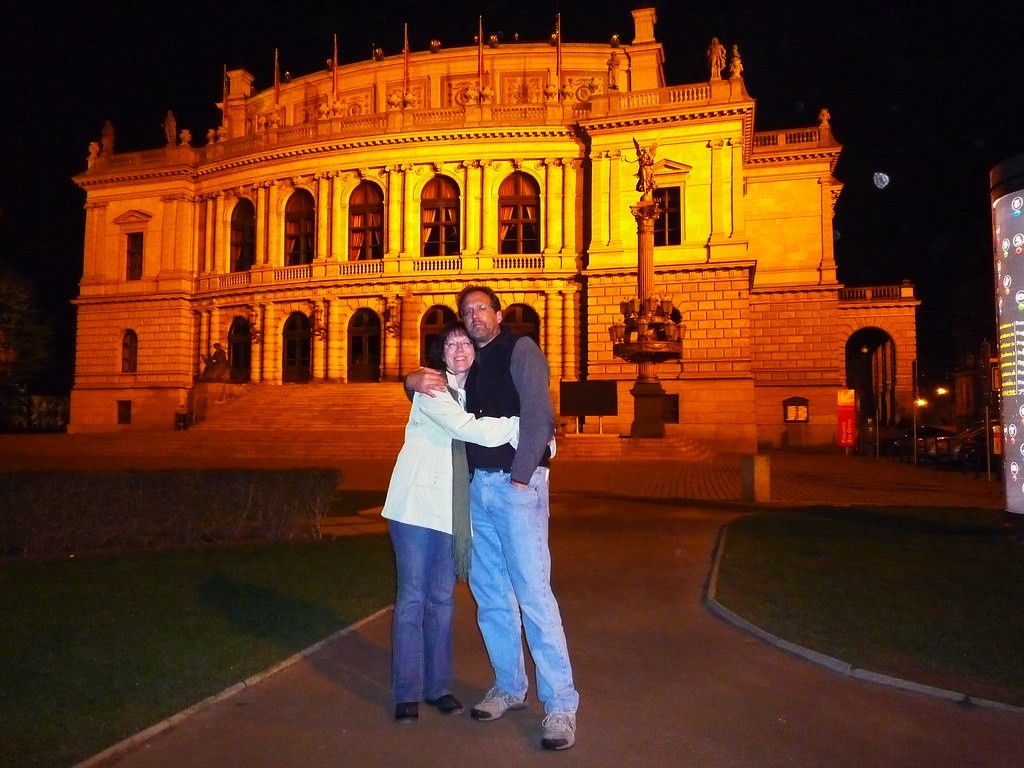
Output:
[444,340,473,349]
[461,304,497,315]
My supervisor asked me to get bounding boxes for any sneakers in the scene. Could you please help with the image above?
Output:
[471,686,528,722]
[426,695,465,716]
[540,712,578,749]
[395,701,420,724]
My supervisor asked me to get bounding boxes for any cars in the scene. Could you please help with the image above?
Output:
[857,416,1002,467]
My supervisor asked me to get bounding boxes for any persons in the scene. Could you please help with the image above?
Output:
[606,51,620,90]
[639,147,652,196]
[199,343,226,381]
[456,284,580,750]
[707,38,726,80]
[160,110,176,144]
[100,121,114,153]
[174,405,191,430]
[380,321,556,725]
